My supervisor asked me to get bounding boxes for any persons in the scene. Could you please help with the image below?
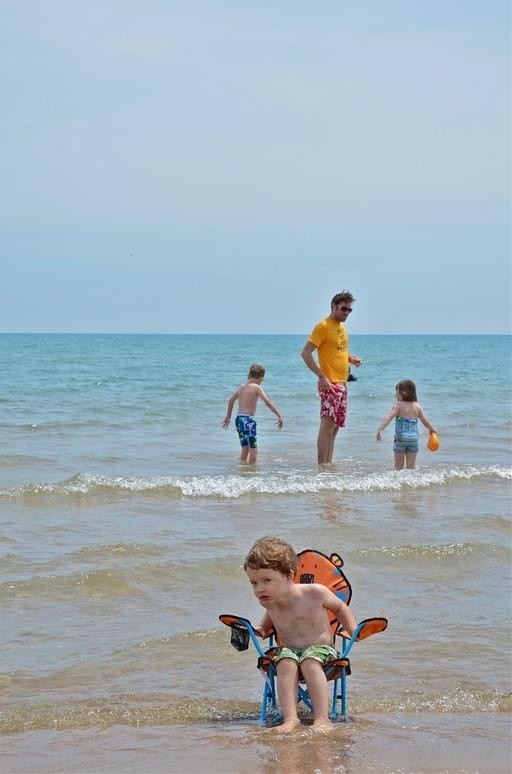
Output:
[375,378,438,467]
[300,289,362,468]
[242,534,360,734]
[220,362,285,465]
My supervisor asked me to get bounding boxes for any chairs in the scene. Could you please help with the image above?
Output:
[219,549,387,725]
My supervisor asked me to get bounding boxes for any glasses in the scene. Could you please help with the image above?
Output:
[337,304,352,313]
